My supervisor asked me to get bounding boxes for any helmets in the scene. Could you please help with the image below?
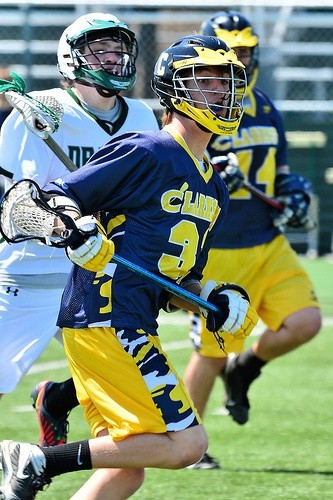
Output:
[150,35,248,136]
[57,13,137,97]
[198,10,260,99]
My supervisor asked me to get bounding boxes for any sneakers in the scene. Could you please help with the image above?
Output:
[220,352,252,425]
[0,440,53,500]
[185,454,219,469]
[30,380,70,446]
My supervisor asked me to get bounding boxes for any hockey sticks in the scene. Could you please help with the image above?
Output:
[1,179,219,314]
[210,163,286,212]
[0,73,79,171]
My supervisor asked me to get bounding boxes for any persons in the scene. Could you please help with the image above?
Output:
[0,13,162,398]
[183,10,321,470]
[0,35,258,500]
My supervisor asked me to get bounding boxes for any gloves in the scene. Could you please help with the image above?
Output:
[199,279,258,340]
[65,214,114,273]
[274,173,312,226]
